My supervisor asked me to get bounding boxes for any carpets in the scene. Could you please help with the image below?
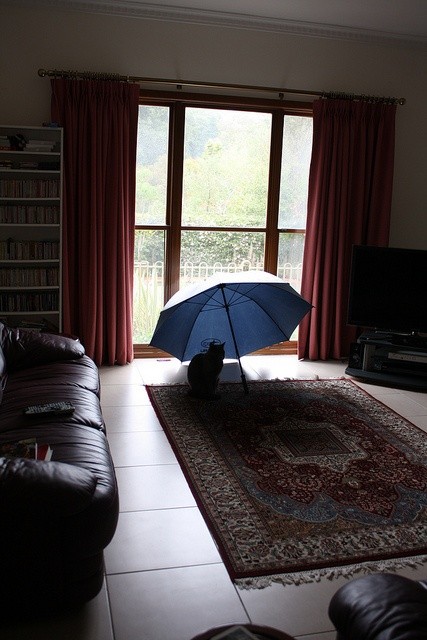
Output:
[145,379,427,589]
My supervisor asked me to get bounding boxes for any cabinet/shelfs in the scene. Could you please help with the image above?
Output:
[0,124,63,335]
[345,329,426,393]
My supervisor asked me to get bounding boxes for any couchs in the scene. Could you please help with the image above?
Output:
[0,322,142,623]
[327,572,427,639]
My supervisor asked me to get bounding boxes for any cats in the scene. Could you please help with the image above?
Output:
[186,338,228,396]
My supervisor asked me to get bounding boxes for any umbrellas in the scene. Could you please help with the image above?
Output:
[148,270,317,394]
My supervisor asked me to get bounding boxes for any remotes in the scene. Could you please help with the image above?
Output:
[18,400,75,416]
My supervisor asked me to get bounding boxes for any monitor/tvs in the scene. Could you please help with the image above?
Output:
[347,243,427,345]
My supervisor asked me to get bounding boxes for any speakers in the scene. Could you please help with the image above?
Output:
[349,342,362,369]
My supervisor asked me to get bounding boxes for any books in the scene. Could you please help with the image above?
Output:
[2,205,60,225]
[2,157,60,170]
[1,239,59,259]
[0,129,60,152]
[0,268,58,286]
[2,438,54,463]
[2,178,60,198]
[0,292,59,310]
[0,317,59,333]
